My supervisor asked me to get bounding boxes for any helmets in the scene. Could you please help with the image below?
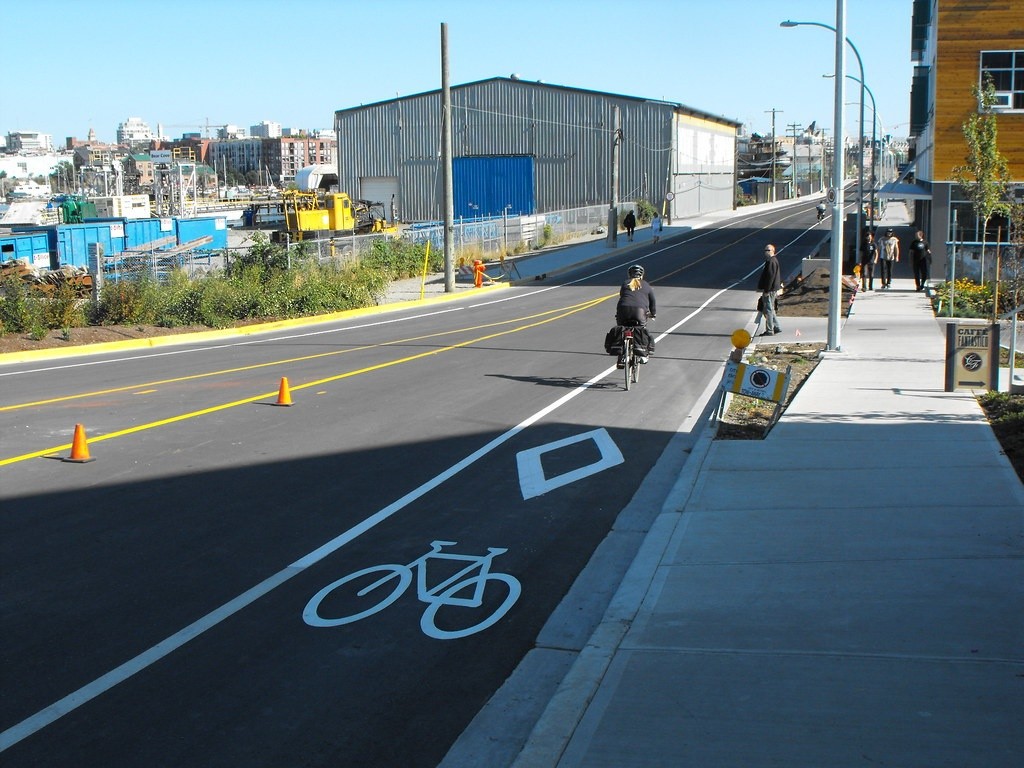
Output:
[627,264,644,278]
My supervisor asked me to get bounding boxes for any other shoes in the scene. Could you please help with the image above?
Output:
[759,332,774,336]
[774,329,782,333]
[861,288,866,292]
[617,360,624,369]
[869,288,873,290]
[648,346,655,352]
[887,284,890,288]
[881,283,886,288]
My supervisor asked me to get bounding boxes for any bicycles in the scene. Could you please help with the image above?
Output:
[615,314,656,390]
[815,208,826,222]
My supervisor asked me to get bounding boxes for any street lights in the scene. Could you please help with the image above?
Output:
[780,19,864,263]
[822,73,874,243]
[843,101,882,220]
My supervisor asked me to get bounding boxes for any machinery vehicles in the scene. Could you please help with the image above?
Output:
[279,188,398,244]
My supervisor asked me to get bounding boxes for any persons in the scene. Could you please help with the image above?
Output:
[797,187,801,199]
[651,212,661,244]
[625,210,636,242]
[859,228,899,291]
[908,230,931,292]
[758,244,783,336]
[815,201,826,222]
[616,265,656,370]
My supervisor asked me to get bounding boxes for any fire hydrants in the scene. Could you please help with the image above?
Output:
[472,259,487,288]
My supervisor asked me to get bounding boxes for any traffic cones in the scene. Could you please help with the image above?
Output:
[274,377,296,406]
[63,422,96,462]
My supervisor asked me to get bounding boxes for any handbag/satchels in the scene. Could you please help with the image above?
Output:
[605,326,626,357]
[624,218,631,227]
[660,226,662,231]
[757,296,778,314]
[633,326,651,357]
